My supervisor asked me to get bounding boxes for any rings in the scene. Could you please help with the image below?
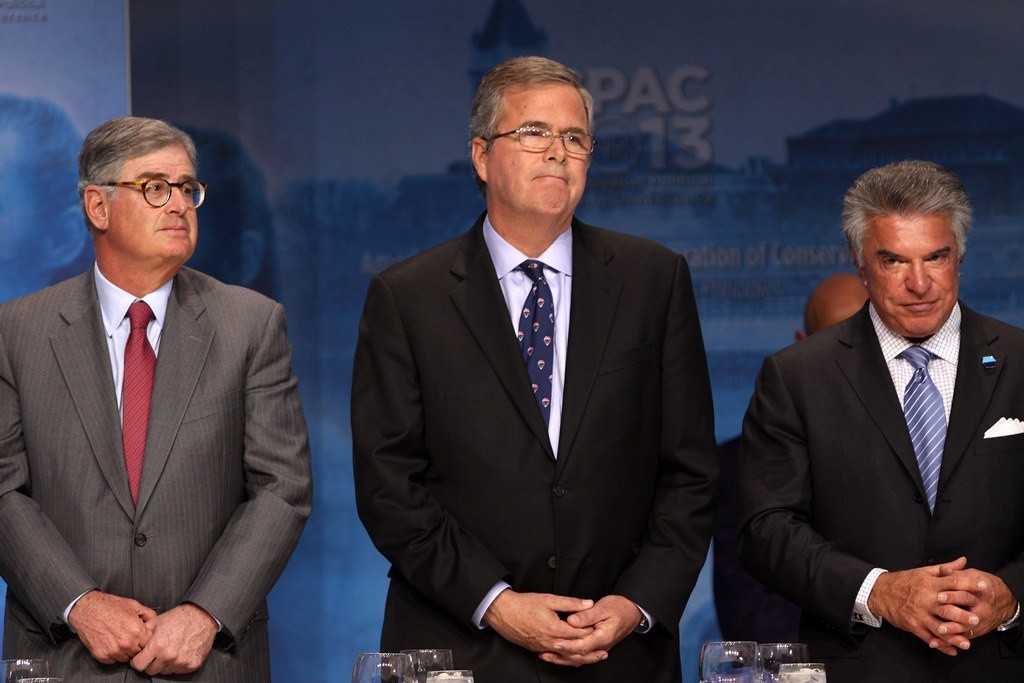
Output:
[969,629,974,638]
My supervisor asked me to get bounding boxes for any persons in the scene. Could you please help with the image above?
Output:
[0,115,314,683]
[350,56,722,683]
[711,160,1023,683]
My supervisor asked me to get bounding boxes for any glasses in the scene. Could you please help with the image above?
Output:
[485,126,597,155]
[93,178,208,209]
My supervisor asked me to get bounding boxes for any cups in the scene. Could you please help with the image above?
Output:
[398,649,453,683]
[758,644,810,683]
[17,678,63,683]
[426,670,474,683]
[352,653,415,683]
[699,642,759,683]
[0,659,49,683]
[778,663,826,683]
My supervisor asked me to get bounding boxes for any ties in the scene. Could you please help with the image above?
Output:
[511,259,559,429]
[900,345,947,516]
[122,300,156,510]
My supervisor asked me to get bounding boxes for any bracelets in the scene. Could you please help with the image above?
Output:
[639,619,648,631]
